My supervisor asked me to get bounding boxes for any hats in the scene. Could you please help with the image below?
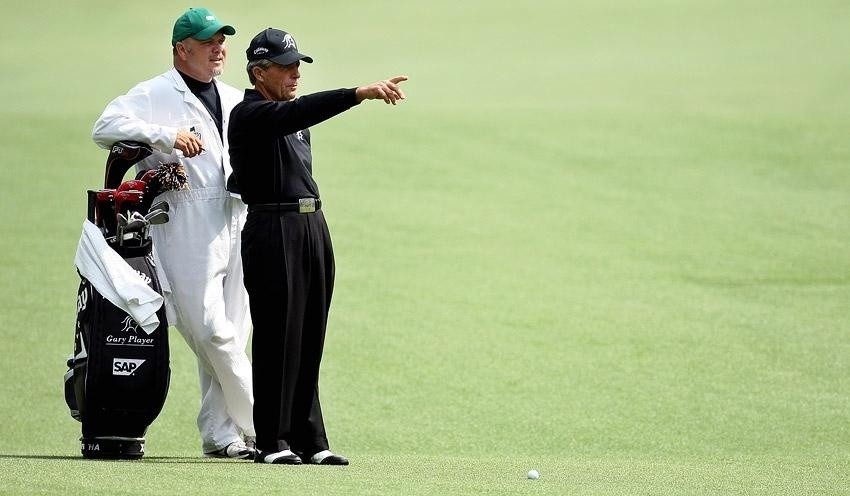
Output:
[247,27,313,66]
[173,8,235,46]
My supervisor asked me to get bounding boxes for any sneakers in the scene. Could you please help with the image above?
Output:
[204,434,256,460]
[254,448,302,464]
[298,449,348,464]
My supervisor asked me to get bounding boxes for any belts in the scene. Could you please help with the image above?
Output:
[252,198,322,212]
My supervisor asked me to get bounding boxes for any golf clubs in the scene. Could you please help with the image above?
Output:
[116,201,170,246]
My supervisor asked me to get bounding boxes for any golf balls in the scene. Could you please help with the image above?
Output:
[527,470,539,479]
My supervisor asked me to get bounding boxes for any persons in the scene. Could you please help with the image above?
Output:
[225,23,410,465]
[92,5,263,463]
